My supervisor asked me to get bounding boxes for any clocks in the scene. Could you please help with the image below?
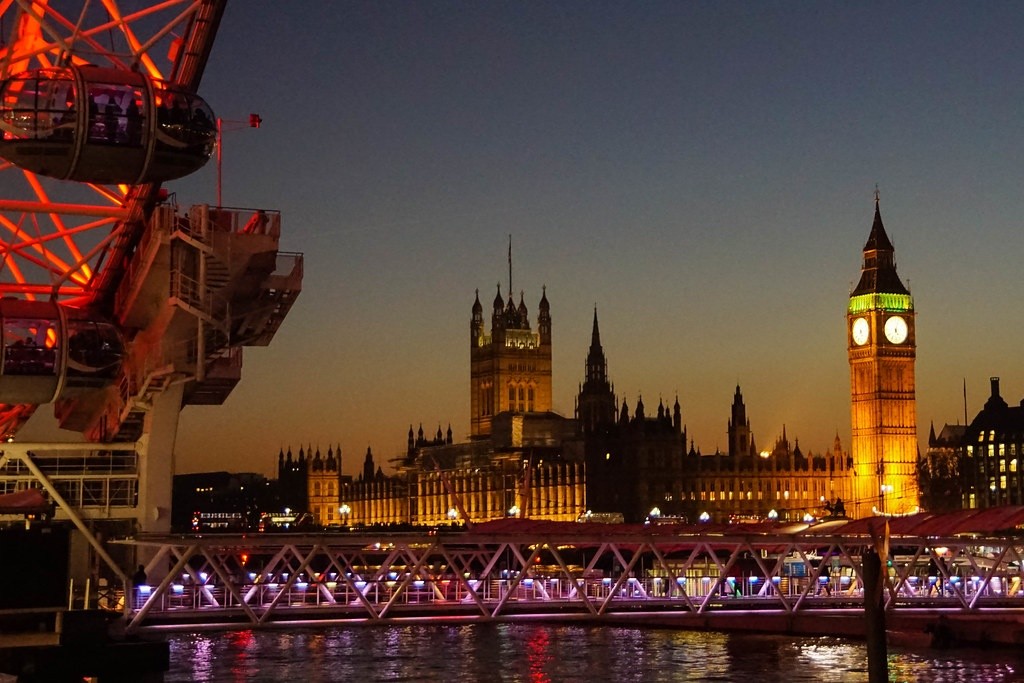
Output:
[852,318,869,345]
[885,316,908,344]
